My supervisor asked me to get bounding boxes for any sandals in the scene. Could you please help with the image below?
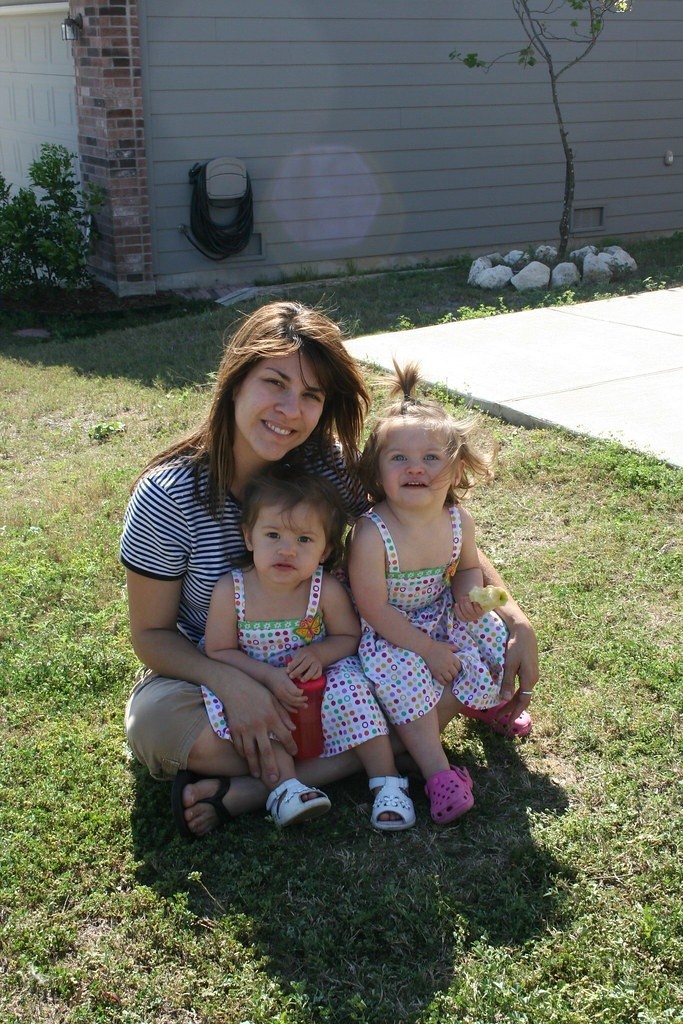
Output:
[424,761,474,825]
[463,696,532,738]
[367,773,416,830]
[265,777,331,830]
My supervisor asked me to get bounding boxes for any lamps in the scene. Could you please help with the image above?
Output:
[61,9,82,40]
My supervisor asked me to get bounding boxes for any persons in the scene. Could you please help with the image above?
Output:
[118,302,540,837]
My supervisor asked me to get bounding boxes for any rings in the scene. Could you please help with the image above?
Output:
[520,691,534,695]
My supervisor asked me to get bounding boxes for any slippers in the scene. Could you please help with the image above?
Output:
[172,767,237,845]
